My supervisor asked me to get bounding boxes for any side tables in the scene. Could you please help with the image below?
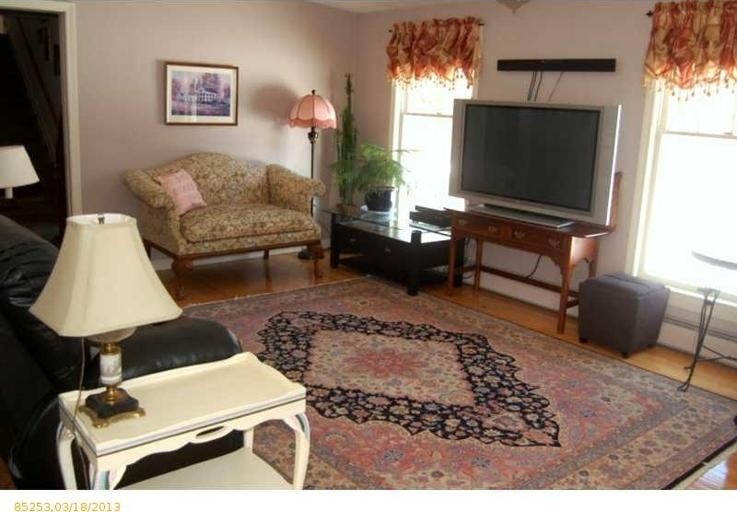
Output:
[54,352,309,490]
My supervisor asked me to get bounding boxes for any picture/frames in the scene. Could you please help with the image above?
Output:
[164,60,240,126]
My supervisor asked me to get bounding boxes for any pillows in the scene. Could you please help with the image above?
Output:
[152,168,207,215]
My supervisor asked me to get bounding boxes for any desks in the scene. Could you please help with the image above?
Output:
[447,201,599,335]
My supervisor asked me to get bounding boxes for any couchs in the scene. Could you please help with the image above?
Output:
[0,212,248,489]
[123,150,327,299]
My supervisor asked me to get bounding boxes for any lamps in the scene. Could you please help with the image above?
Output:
[289,88,338,261]
[0,144,40,199]
[29,212,184,429]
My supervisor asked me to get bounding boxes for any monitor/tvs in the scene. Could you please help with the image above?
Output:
[448,99,622,229]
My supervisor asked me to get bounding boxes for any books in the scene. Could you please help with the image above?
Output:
[409,222,452,233]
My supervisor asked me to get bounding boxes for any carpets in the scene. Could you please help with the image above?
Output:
[183,274,736,490]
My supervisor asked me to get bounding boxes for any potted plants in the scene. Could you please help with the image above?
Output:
[328,145,415,211]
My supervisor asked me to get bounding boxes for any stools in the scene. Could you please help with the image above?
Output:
[577,272,671,359]
